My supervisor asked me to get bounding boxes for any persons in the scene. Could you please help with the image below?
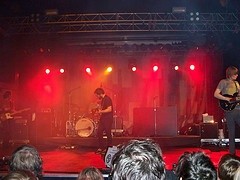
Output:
[78,166,104,180]
[0,91,14,148]
[2,144,42,180]
[110,139,240,180]
[93,87,113,154]
[214,66,240,154]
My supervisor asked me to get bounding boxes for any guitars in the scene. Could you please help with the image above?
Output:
[219,90,240,112]
[0,107,32,123]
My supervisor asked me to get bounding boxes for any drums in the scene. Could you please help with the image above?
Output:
[75,117,97,138]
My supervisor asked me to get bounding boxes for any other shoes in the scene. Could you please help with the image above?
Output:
[100,150,106,154]
[96,149,102,154]
